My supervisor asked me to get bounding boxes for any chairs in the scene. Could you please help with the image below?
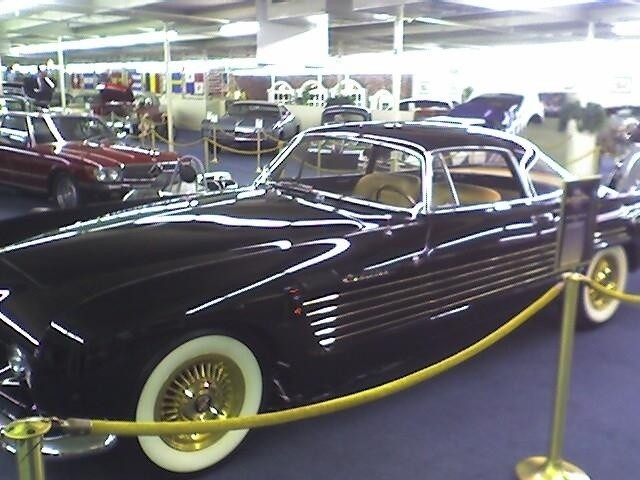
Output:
[431,183,501,207]
[352,171,421,209]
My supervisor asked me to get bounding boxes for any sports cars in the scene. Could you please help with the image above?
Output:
[1,121,639,480]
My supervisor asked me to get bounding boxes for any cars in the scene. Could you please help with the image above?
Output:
[384,84,640,140]
[206,98,302,156]
[0,80,178,145]
[0,110,195,211]
[610,141,640,270]
[317,105,372,124]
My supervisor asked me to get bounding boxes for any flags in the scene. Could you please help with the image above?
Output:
[66,73,203,95]
